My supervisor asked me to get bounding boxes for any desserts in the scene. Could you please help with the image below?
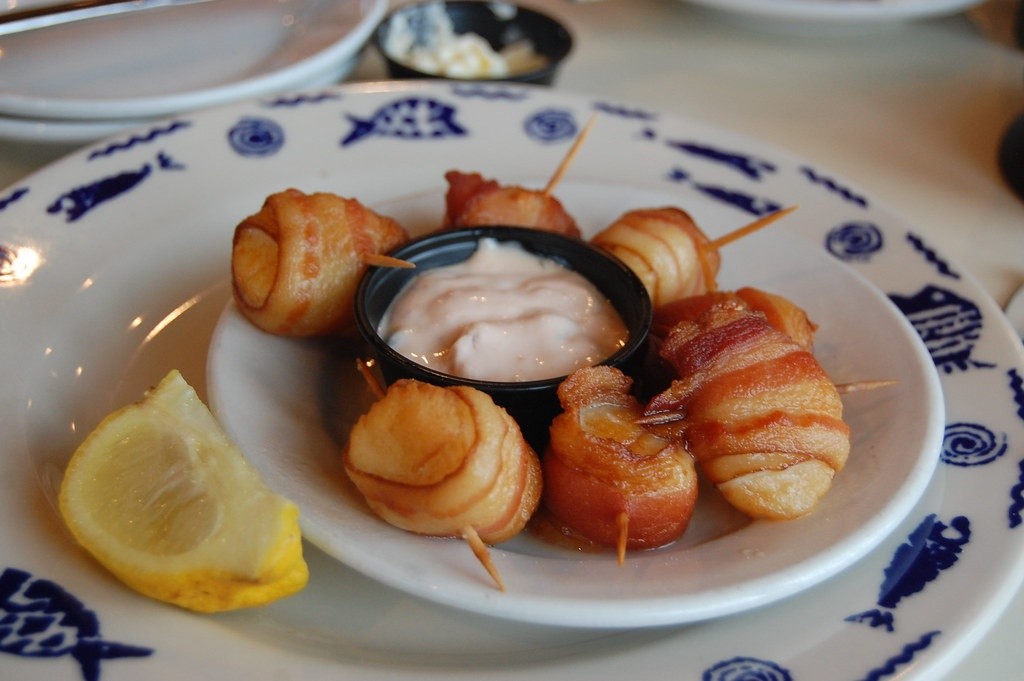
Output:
[379,237,626,381]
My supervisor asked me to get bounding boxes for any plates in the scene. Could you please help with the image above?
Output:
[0,0,387,143]
[206,181,946,630]
[0,81,1024,681]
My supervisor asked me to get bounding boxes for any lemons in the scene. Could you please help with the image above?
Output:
[59,370,308,611]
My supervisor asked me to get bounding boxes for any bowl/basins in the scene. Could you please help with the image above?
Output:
[355,224,653,444]
[373,0,573,88]
